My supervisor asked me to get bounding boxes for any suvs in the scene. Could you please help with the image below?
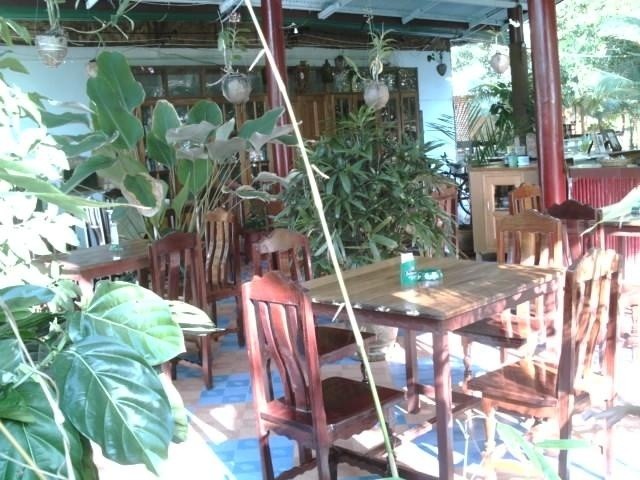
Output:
[562,129,626,171]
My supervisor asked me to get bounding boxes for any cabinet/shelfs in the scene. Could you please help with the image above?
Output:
[467,157,575,261]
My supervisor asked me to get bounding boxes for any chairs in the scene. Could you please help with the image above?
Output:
[458,246,624,480]
[251,228,379,385]
[39,203,245,391]
[453,181,639,394]
[242,272,408,480]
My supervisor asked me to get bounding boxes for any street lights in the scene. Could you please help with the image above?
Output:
[221,69,258,224]
[362,80,391,176]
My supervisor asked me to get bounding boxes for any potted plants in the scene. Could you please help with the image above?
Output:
[340,21,398,110]
[203,11,253,105]
[30,0,139,77]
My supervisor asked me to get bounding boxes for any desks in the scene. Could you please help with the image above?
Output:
[300,255,566,479]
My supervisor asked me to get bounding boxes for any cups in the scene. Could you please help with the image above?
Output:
[504,145,530,167]
[399,257,416,285]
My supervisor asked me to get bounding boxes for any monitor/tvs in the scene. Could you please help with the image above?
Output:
[605,128,622,151]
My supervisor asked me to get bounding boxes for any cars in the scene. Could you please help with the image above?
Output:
[65,187,119,264]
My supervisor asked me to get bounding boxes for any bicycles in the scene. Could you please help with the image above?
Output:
[438,152,500,217]
